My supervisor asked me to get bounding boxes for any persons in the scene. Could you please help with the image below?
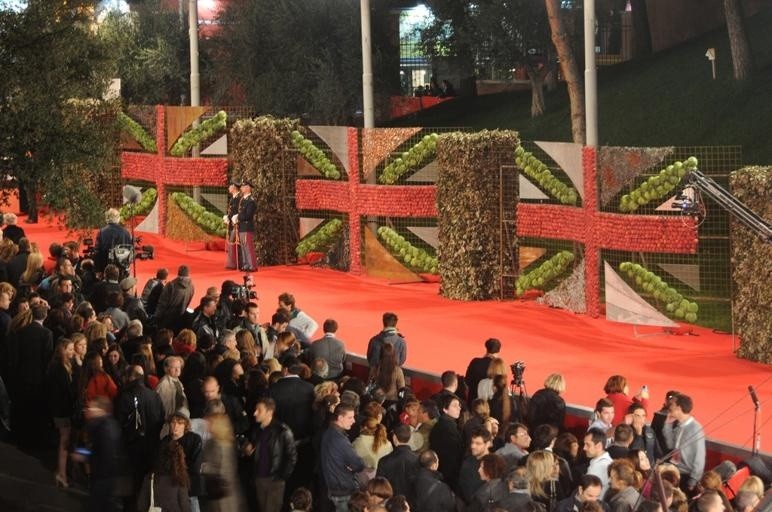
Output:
[231,179,258,272]
[414,79,456,97]
[1,207,224,512]
[224,280,771,512]
[222,180,241,271]
[24,148,38,224]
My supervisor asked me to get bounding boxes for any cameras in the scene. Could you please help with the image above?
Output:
[510,360,526,380]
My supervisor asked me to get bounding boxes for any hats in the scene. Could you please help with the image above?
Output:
[120,278,137,290]
[168,406,191,424]
[230,180,240,186]
[241,179,254,188]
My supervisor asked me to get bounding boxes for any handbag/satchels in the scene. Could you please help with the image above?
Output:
[121,409,144,447]
[289,312,318,339]
[149,506,161,512]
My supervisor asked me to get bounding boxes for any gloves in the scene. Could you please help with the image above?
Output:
[231,215,239,224]
[222,215,231,224]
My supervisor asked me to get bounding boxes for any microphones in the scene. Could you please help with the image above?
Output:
[123,185,148,206]
[748,385,760,408]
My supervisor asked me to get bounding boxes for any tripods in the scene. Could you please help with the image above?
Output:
[510,379,528,421]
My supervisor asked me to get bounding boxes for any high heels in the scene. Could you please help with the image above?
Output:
[55,472,69,488]
